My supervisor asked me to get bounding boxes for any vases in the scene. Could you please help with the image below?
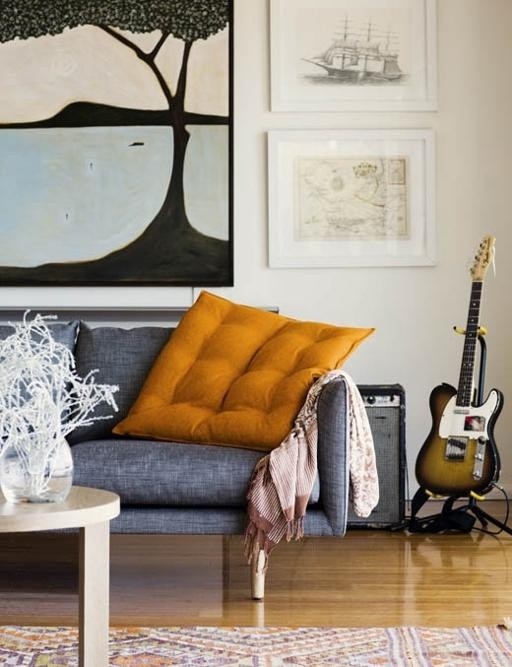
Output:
[0,436,74,503]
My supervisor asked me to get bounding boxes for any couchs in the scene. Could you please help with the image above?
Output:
[0,379,350,599]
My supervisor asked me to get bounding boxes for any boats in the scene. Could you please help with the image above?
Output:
[302,15,402,81]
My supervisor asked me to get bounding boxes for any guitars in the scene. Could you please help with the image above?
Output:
[416,233,504,497]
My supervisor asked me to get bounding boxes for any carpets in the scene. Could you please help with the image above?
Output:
[0,619,511,667]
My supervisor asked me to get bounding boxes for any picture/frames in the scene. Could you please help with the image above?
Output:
[268,130,437,268]
[270,0,438,113]
[0,0,234,287]
